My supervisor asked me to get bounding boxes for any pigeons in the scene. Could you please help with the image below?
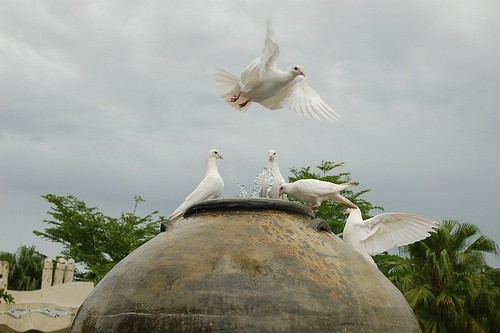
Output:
[278,178,357,211]
[341,205,440,269]
[167,148,224,221]
[215,18,341,121]
[258,150,287,200]
